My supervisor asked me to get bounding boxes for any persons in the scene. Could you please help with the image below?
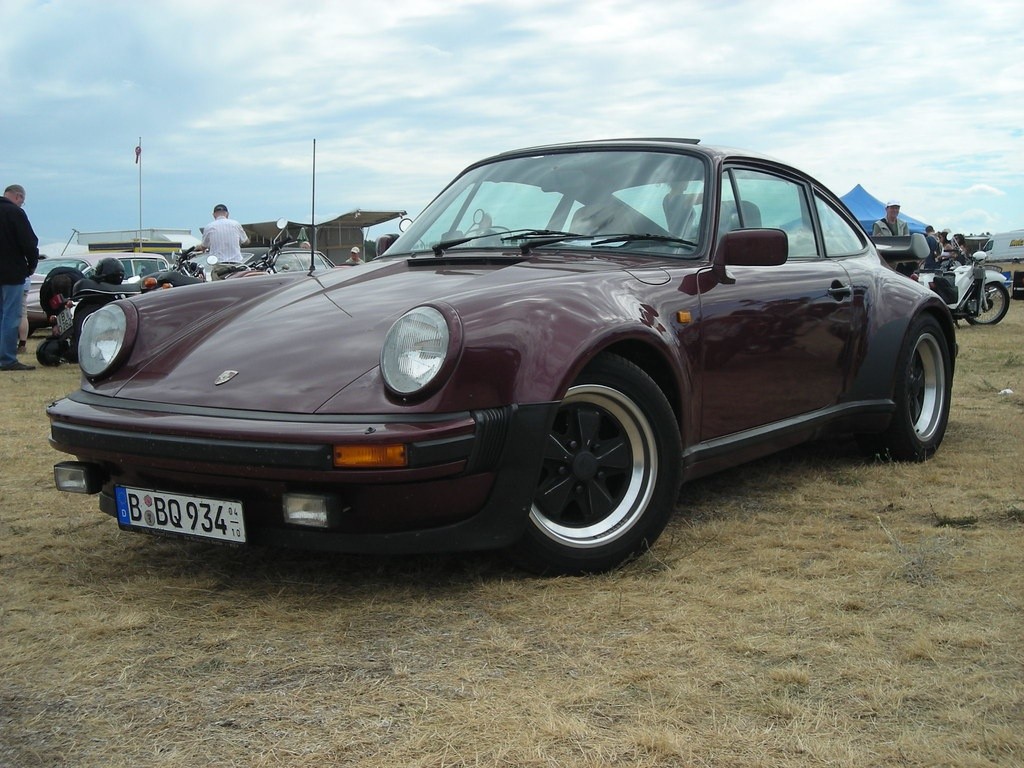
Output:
[873,202,909,236]
[663,181,704,237]
[0,186,39,373]
[40,267,86,328]
[195,204,250,283]
[469,213,503,246]
[346,247,365,264]
[924,225,966,261]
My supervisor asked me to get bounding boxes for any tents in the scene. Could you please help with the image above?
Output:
[779,183,926,234]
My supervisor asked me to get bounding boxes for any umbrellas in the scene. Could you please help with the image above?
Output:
[297,227,310,241]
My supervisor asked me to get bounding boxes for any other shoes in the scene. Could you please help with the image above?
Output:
[17,345,27,354]
[0,360,36,371]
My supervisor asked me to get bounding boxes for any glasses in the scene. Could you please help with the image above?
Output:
[21,200,26,208]
[939,236,945,239]
[955,237,961,239]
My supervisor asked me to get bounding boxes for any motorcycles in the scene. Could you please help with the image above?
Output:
[908,235,1010,329]
[36,208,513,367]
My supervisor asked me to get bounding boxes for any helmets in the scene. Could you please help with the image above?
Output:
[90,257,125,285]
[36,339,62,367]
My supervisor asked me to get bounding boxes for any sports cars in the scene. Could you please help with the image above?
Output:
[43,137,960,583]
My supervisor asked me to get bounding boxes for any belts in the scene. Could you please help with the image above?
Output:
[221,261,238,265]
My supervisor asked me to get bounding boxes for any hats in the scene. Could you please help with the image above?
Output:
[214,204,228,212]
[886,200,900,207]
[351,246,361,254]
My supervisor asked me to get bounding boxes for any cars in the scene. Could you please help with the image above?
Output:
[23,252,169,338]
[137,246,338,290]
